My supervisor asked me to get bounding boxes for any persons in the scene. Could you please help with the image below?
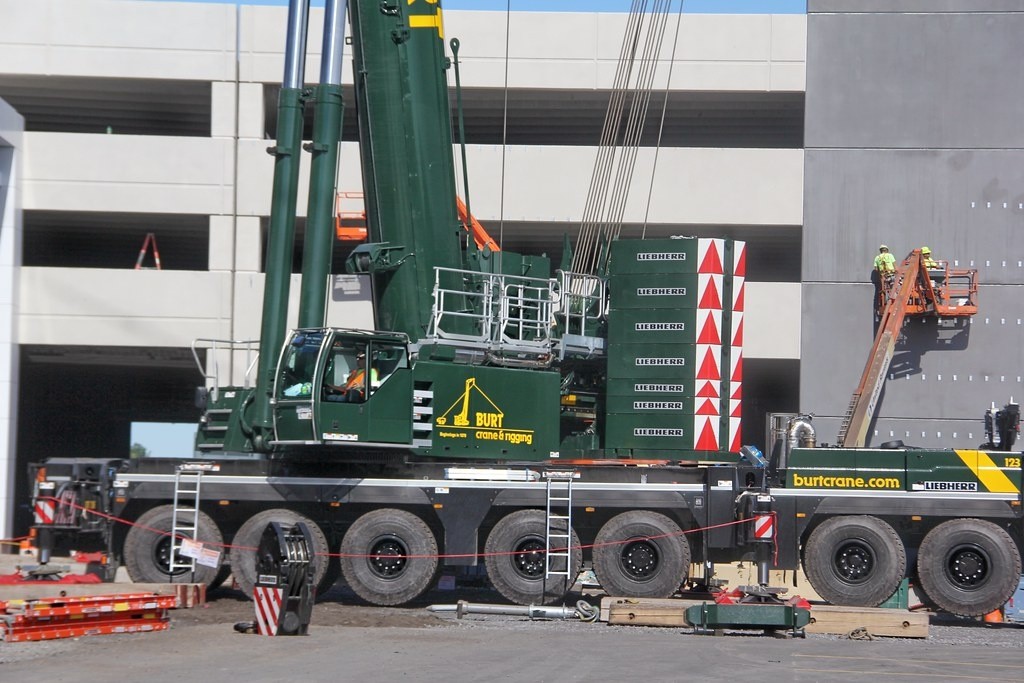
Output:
[921,247,937,270]
[328,351,378,402]
[874,245,898,302]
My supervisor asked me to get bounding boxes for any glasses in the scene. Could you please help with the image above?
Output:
[356,357,363,360]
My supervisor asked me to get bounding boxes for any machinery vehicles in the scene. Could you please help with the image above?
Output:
[833,247,979,447]
[107,2,1024,625]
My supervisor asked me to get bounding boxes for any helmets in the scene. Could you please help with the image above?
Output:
[879,244,889,251]
[921,246,932,254]
